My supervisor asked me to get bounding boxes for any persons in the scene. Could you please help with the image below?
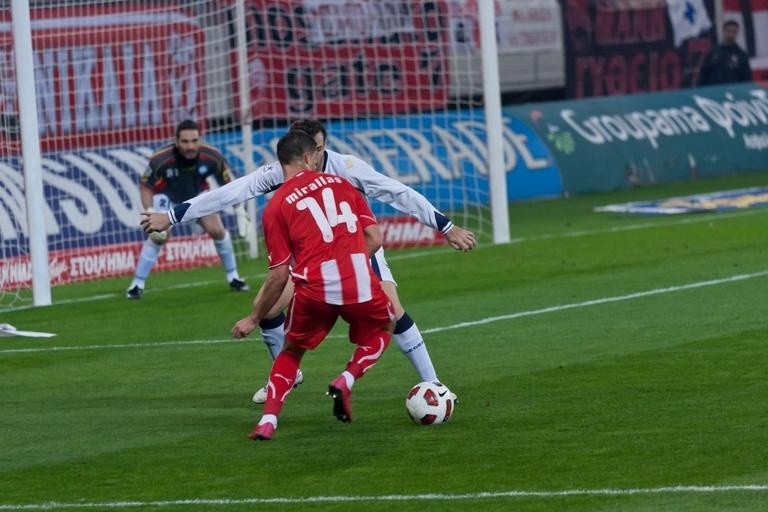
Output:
[125,118,250,299]
[696,21,753,86]
[140,123,478,402]
[231,130,395,439]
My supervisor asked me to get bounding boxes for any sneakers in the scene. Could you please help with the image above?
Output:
[252,369,303,404]
[452,393,459,404]
[248,422,274,440]
[326,374,352,424]
[126,282,143,299]
[230,278,250,291]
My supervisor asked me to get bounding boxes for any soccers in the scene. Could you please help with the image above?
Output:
[406,382,454,425]
